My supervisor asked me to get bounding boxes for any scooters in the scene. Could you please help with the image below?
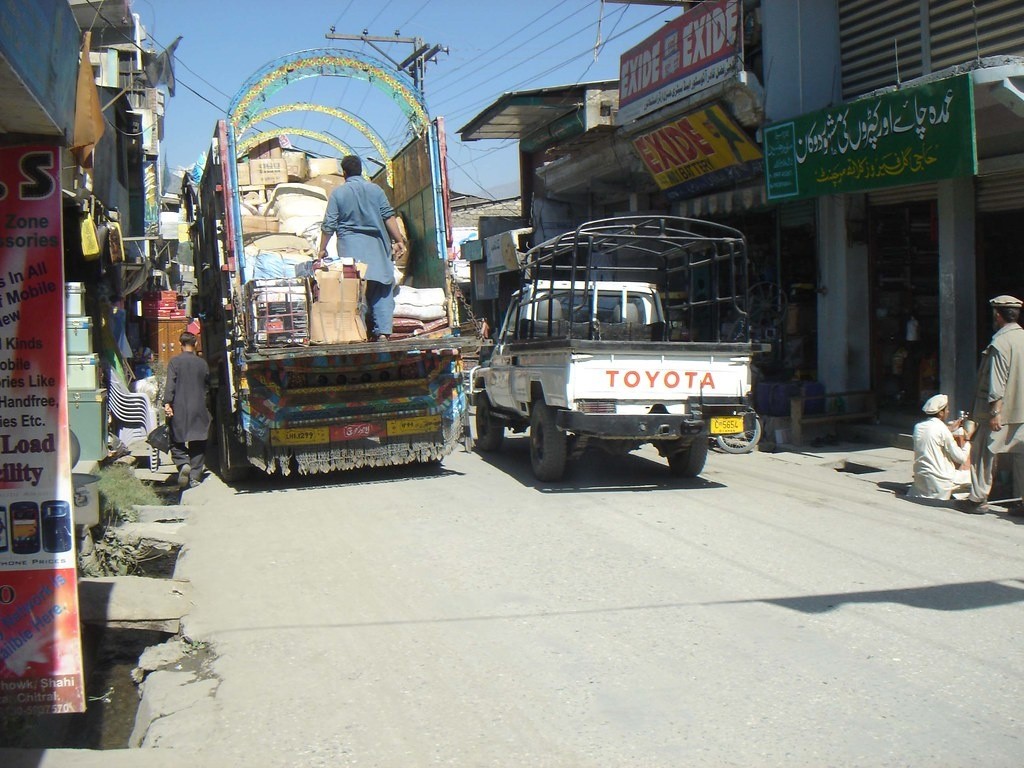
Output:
[717,406,761,454]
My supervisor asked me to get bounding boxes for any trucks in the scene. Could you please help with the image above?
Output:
[180,47,467,485]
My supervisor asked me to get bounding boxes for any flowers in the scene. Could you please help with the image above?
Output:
[829,387,849,416]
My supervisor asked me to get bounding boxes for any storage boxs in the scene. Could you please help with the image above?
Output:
[236,138,410,344]
[64,281,108,460]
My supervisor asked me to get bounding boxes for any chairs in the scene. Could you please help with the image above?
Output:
[612,302,640,324]
[537,296,563,321]
[108,367,157,472]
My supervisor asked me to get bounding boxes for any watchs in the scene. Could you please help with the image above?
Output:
[988,410,1001,417]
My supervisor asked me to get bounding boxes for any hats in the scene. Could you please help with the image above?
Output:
[989,295,1023,307]
[922,394,948,414]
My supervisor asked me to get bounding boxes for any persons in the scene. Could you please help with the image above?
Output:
[109,295,134,358]
[145,332,214,490]
[906,394,981,501]
[318,155,407,342]
[950,294,1024,518]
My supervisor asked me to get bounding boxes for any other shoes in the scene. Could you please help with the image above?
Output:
[190,479,201,488]
[177,464,191,488]
[1008,504,1024,516]
[953,499,989,514]
[377,335,388,341]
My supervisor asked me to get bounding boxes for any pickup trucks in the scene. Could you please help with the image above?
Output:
[468,282,771,480]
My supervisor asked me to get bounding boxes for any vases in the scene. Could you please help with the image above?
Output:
[837,419,861,442]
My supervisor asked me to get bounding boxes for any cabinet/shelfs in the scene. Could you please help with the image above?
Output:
[789,389,882,446]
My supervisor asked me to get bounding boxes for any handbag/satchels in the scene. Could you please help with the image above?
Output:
[146,416,175,454]
